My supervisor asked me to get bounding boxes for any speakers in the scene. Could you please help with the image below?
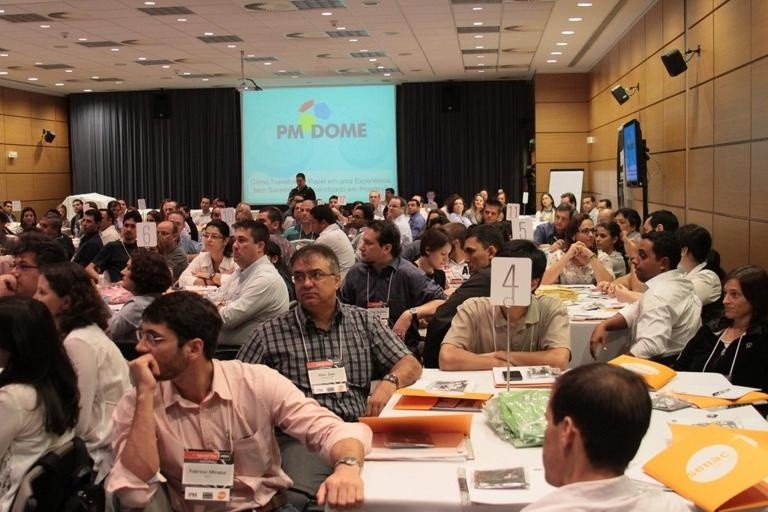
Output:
[611,85,629,105]
[675,77,678,79]
[661,48,688,78]
[45,131,56,143]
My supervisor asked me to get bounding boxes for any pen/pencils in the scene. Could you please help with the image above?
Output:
[714,388,730,396]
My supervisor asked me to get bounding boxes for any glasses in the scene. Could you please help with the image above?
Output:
[10,261,42,274]
[134,328,183,349]
[289,269,338,283]
[574,227,596,234]
[203,232,227,240]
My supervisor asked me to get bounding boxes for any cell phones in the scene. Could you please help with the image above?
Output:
[502,370,523,381]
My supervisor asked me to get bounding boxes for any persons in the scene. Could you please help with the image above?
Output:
[560,193,579,218]
[210,197,255,224]
[369,192,385,215]
[100,208,121,245]
[104,247,174,341]
[671,265,767,392]
[56,203,69,231]
[281,172,317,239]
[84,208,142,281]
[533,203,573,242]
[385,189,394,207]
[352,201,364,214]
[118,199,127,214]
[597,199,612,211]
[256,206,297,266]
[438,239,573,371]
[309,204,355,281]
[389,197,413,244]
[613,207,643,257]
[412,227,450,292]
[328,195,350,223]
[535,192,557,223]
[597,224,722,304]
[33,263,135,489]
[0,201,18,223]
[70,208,104,263]
[192,196,213,231]
[107,200,123,229]
[179,220,241,295]
[178,202,198,241]
[597,210,614,223]
[409,190,510,242]
[39,207,74,259]
[156,221,188,282]
[382,207,389,221]
[70,198,84,233]
[420,223,508,370]
[607,209,681,297]
[0,232,65,294]
[232,243,422,512]
[581,195,599,227]
[540,214,614,286]
[17,208,37,231]
[349,204,375,260]
[263,240,295,302]
[589,230,703,361]
[336,221,448,342]
[128,199,179,221]
[204,222,290,344]
[1,296,80,512]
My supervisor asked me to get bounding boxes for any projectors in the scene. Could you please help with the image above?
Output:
[236,86,257,92]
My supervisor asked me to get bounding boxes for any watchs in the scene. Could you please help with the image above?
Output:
[587,253,597,260]
[333,455,364,471]
[384,374,399,386]
[104,290,373,511]
[409,306,420,322]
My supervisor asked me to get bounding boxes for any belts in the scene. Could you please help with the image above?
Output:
[254,491,289,512]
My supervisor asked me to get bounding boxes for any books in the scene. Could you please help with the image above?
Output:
[393,388,489,415]
[644,421,767,512]
[492,366,558,388]
[359,414,471,462]
[606,353,676,391]
[520,363,696,512]
[652,379,767,411]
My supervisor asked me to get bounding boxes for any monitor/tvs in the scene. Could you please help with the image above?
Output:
[622,117,647,187]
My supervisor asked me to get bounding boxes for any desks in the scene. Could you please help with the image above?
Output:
[535,284,633,364]
[324,367,768,512]
